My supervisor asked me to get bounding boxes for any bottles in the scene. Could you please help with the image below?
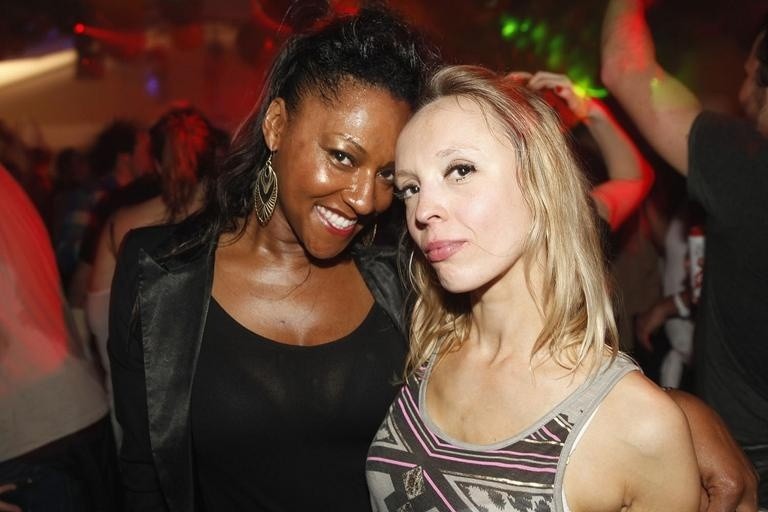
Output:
[686,227,705,300]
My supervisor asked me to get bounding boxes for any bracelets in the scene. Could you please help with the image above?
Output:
[659,385,674,393]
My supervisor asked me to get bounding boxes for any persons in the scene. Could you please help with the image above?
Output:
[367,67,700,509]
[599,0,767,510]
[0,102,706,457]
[0,484,24,511]
[0,2,761,511]
